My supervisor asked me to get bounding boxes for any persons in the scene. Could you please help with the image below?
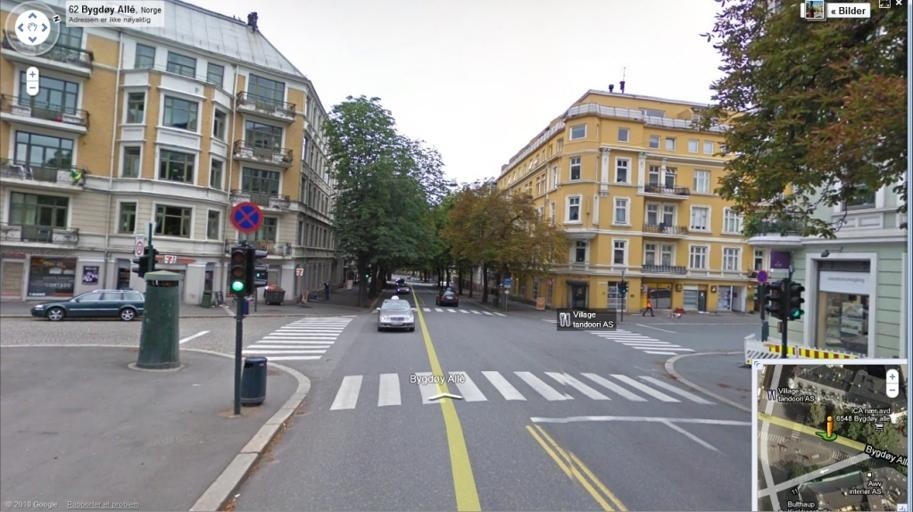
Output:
[323,282,330,301]
[642,295,655,317]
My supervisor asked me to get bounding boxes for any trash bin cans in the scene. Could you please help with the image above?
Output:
[202,291,211,307]
[240,357,267,406]
